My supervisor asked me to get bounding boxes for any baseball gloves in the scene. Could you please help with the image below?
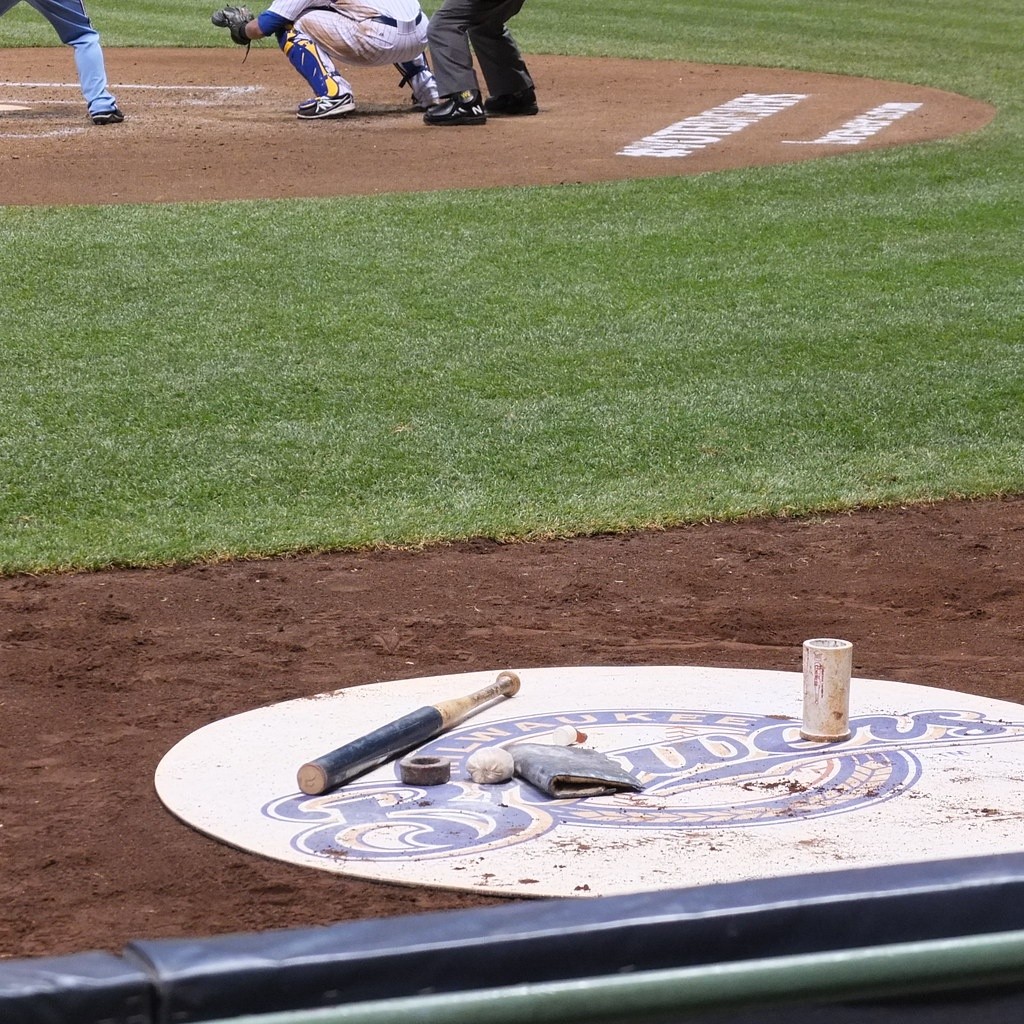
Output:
[211,3,255,44]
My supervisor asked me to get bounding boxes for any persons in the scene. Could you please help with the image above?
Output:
[0,0,125,126]
[423,0,538,126]
[231,0,442,120]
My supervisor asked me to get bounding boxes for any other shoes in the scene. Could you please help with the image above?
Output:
[484,88,539,115]
[423,90,487,125]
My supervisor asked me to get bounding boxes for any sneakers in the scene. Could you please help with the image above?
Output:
[92,104,124,124]
[296,93,356,119]
[411,93,440,107]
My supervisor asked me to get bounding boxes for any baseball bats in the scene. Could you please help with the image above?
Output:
[297,671,521,795]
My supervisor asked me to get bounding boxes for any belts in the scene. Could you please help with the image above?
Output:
[371,9,422,28]
